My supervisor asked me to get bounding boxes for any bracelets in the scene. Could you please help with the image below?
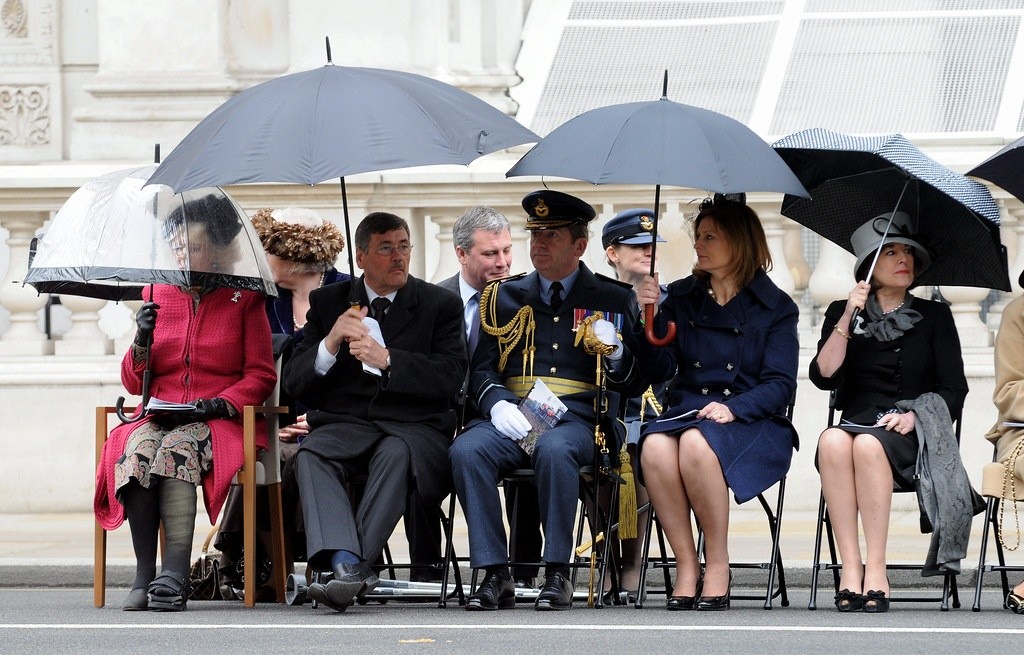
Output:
[833,324,852,341]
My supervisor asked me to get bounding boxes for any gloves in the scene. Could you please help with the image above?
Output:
[594,318,623,360]
[187,397,221,420]
[488,399,532,442]
[134,302,160,348]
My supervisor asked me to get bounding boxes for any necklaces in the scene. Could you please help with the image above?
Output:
[883,301,904,315]
[293,272,325,329]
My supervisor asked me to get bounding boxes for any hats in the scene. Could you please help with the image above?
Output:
[850,212,931,285]
[250,206,346,263]
[521,189,596,230]
[601,209,667,250]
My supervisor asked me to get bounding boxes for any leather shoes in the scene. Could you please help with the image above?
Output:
[534,569,573,610]
[325,562,380,605]
[467,573,516,609]
[308,574,352,612]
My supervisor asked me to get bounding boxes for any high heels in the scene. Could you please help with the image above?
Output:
[666,563,703,610]
[698,569,732,611]
[862,575,890,612]
[596,568,621,605]
[834,575,863,612]
[622,566,646,601]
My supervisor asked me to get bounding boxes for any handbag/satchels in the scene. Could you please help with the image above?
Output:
[187,525,226,601]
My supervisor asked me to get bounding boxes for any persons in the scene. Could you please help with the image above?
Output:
[447,189,650,611]
[282,211,469,613]
[215,207,361,603]
[404,204,543,590]
[580,208,680,605]
[94,194,278,610]
[630,193,799,611]
[984,271,1024,615]
[809,211,970,613]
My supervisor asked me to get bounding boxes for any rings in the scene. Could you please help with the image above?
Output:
[715,412,721,419]
[897,424,903,428]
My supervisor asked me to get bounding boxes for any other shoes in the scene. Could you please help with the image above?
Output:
[123,575,155,612]
[1005,580,1024,614]
[146,571,187,610]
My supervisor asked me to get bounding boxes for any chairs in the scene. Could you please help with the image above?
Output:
[636,383,796,610]
[972,445,1024,612]
[94,334,289,606]
[470,398,629,609]
[310,382,465,608]
[808,390,963,610]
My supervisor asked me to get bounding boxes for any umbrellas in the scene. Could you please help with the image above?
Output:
[964,134,1024,205]
[767,127,1013,334]
[138,35,543,308]
[505,68,814,347]
[21,143,277,424]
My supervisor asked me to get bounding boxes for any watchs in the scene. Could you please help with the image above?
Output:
[383,355,392,374]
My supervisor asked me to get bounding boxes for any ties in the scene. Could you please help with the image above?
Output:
[371,297,392,327]
[467,294,481,366]
[550,281,565,311]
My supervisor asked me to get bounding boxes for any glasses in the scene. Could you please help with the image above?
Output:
[366,243,413,256]
